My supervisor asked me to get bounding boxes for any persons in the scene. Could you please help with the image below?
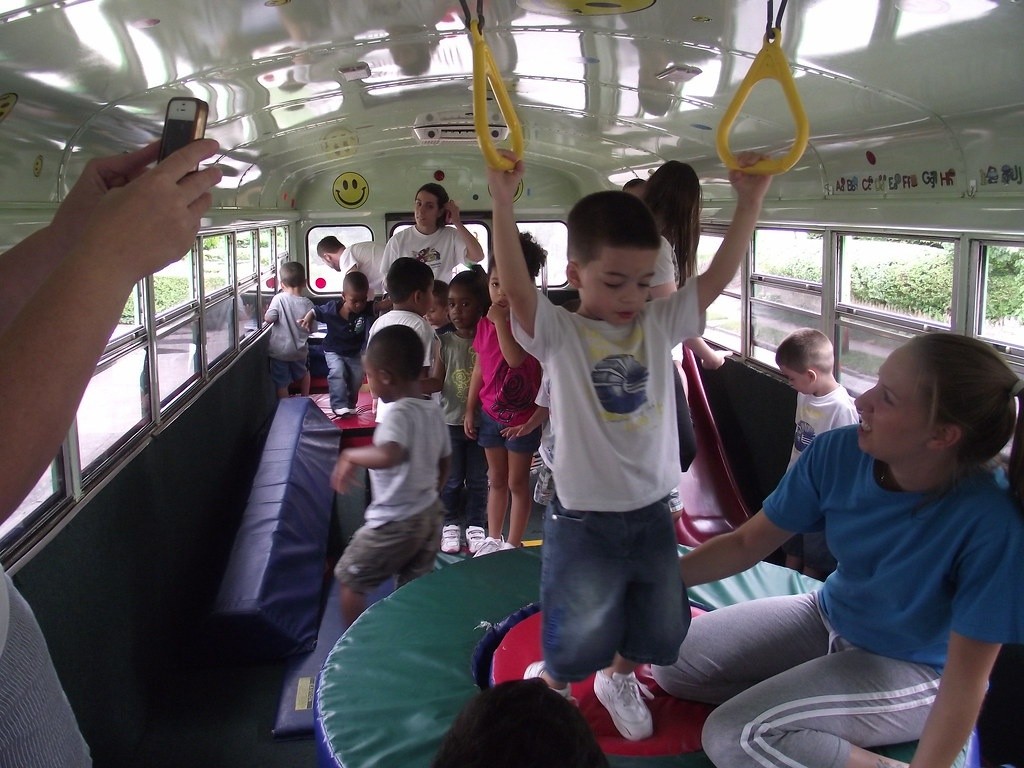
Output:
[0,137,225,765]
[380,182,486,290]
[429,676,611,768]
[420,265,495,555]
[638,158,737,472]
[364,257,439,429]
[622,178,647,200]
[295,272,395,417]
[263,261,318,400]
[329,324,454,632]
[358,1,449,75]
[317,236,393,318]
[619,0,727,118]
[485,144,774,744]
[774,328,861,584]
[465,231,551,559]
[647,333,1023,768]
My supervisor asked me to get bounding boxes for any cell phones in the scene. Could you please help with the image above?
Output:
[153,97,208,185]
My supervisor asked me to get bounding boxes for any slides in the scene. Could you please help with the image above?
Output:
[660,340,754,547]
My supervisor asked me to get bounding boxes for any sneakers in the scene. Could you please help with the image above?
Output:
[473,535,525,558]
[441,523,461,552]
[333,407,357,416]
[464,526,487,552]
[593,670,653,741]
[525,660,572,706]
